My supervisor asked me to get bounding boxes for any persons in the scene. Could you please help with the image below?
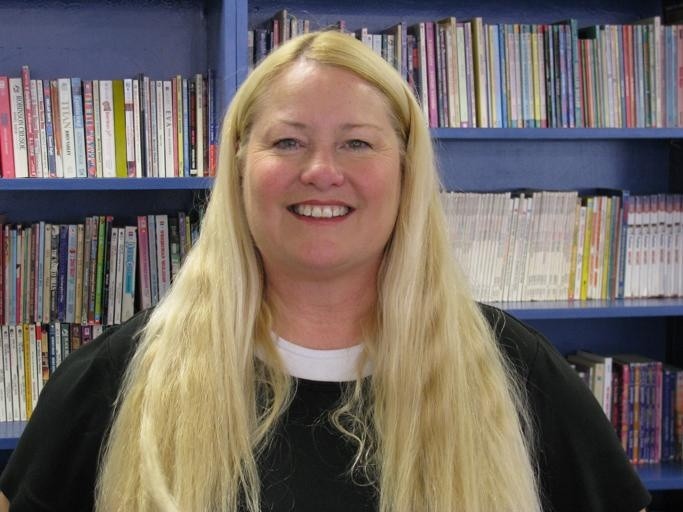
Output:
[1,31,652,512]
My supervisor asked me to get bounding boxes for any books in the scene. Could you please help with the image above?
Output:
[0,62,215,178]
[441,187,683,469]
[249,10,682,128]
[0,208,204,423]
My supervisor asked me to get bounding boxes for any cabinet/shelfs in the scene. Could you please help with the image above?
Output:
[238,0,682,511]
[0,1,235,466]
